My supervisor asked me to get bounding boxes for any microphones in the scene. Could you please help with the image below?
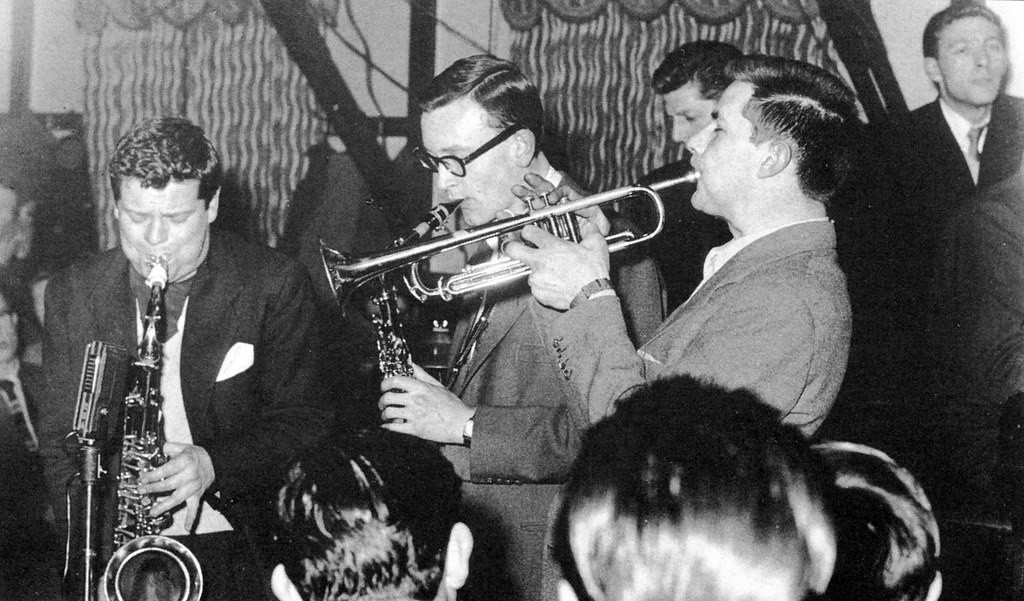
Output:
[72,341,129,448]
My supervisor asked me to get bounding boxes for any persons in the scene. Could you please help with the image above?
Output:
[622,36,759,317]
[807,441,950,601]
[543,374,852,601]
[0,112,97,600]
[850,2,1024,450]
[890,154,1024,600]
[35,113,344,601]
[295,118,409,436]
[376,50,670,601]
[267,424,475,601]
[489,50,863,454]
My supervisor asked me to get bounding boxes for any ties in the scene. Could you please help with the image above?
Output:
[965,128,983,186]
[0,379,36,451]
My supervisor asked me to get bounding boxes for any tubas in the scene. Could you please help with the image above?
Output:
[319,168,703,306]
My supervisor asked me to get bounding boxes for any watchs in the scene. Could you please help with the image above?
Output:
[463,413,478,450]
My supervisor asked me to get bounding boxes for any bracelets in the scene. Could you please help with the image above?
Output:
[568,277,615,312]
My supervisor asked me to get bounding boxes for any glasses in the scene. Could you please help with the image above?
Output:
[414,123,524,178]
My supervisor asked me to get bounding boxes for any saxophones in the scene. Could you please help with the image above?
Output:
[363,195,463,423]
[100,253,203,601]
